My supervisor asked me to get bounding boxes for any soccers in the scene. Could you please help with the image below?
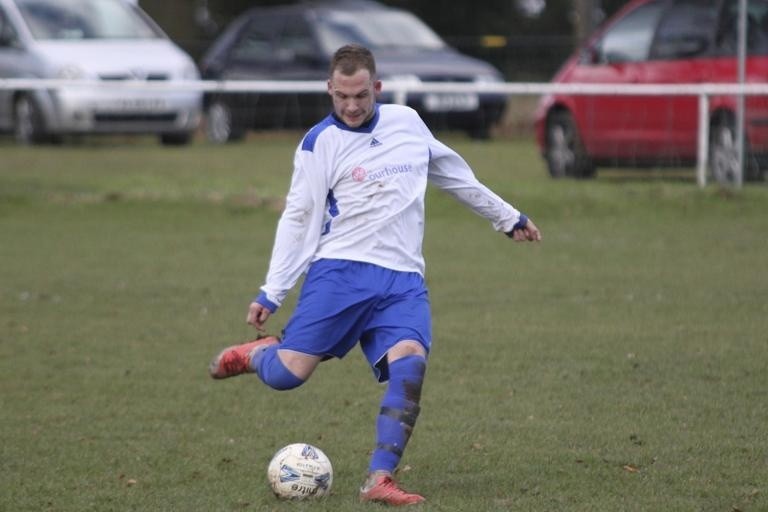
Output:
[267,443,334,501]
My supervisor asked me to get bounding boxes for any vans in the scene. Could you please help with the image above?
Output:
[0,1,208,148]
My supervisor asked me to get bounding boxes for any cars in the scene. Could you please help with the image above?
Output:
[530,1,766,196]
[194,1,514,145]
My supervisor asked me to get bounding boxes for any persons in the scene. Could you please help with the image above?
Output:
[207,43,540,506]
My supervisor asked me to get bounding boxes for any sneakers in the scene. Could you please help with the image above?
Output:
[210,336,281,379]
[359,472,425,505]
